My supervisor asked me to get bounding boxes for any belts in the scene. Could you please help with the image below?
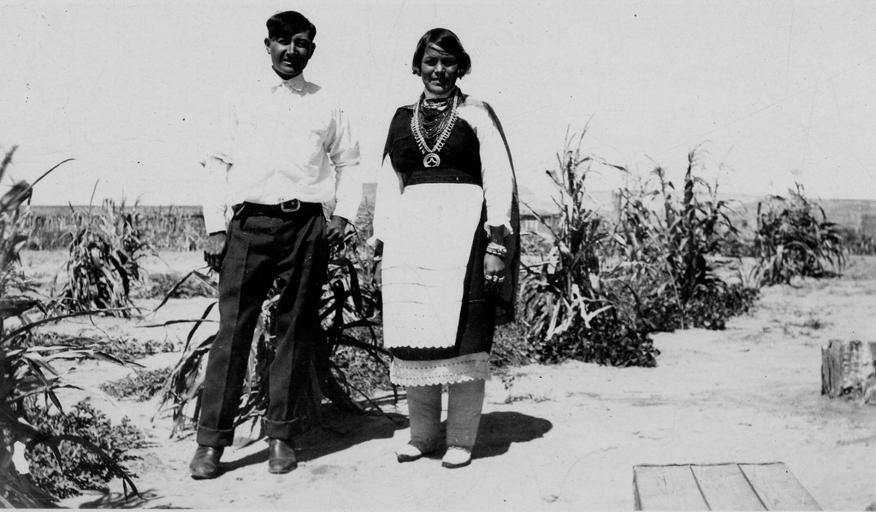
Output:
[232,199,323,218]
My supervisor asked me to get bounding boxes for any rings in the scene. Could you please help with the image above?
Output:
[373,283,377,288]
[486,274,506,283]
[378,283,382,287]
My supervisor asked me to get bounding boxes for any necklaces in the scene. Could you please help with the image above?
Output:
[411,86,460,169]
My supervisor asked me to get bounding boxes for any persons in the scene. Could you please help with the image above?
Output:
[189,11,363,479]
[373,28,521,468]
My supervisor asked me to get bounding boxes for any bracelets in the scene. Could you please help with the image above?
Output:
[486,241,508,258]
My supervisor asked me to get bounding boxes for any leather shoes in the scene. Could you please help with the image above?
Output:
[189,439,295,480]
[396,445,473,467]
